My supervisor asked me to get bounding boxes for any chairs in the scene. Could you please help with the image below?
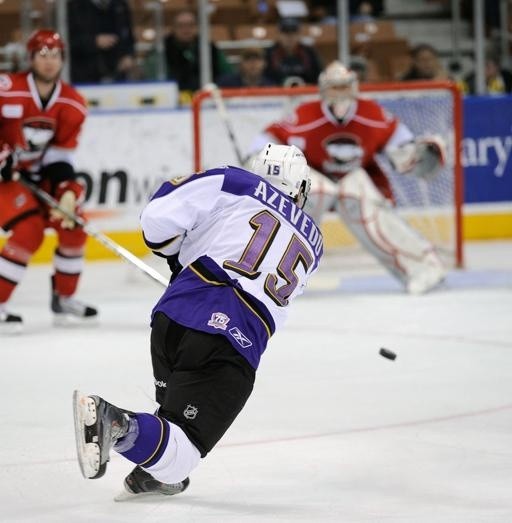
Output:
[0,0,422,93]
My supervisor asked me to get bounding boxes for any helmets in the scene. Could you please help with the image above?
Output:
[27,30,64,62]
[253,142,311,209]
[318,61,358,104]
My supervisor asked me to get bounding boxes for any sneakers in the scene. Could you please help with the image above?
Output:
[51,275,96,315]
[79,394,136,478]
[0,307,20,321]
[124,464,190,495]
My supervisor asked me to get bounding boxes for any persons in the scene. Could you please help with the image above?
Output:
[0,31,100,324]
[306,0,385,23]
[165,12,221,93]
[84,143,324,495]
[67,0,134,83]
[221,18,324,88]
[244,58,458,294]
[402,0,511,96]
